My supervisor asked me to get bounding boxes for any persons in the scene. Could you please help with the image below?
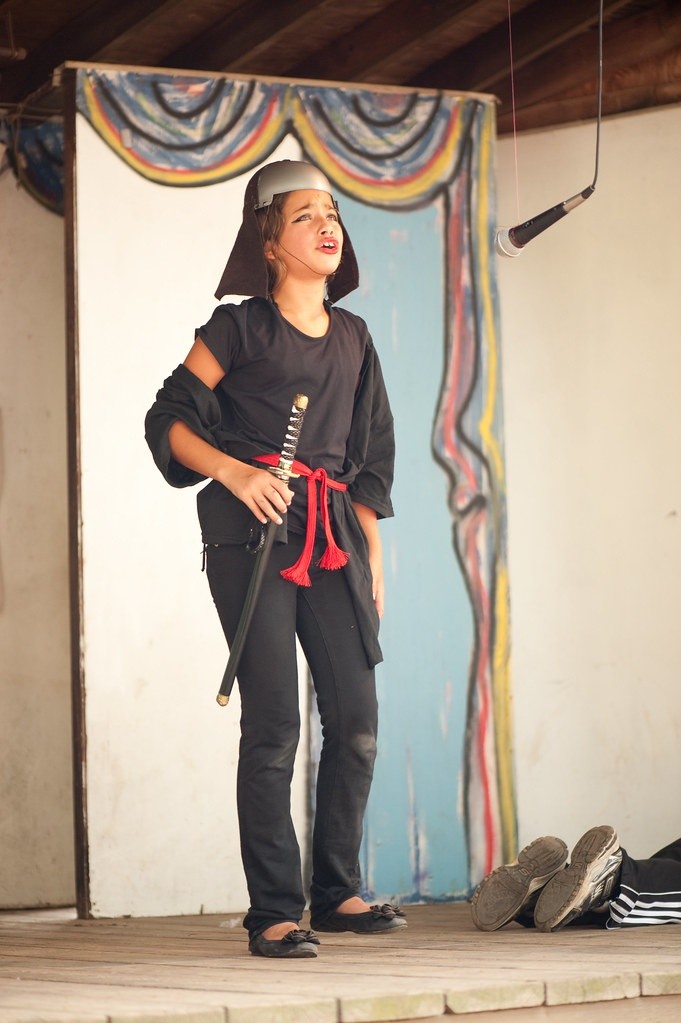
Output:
[472,825,681,934]
[144,161,407,957]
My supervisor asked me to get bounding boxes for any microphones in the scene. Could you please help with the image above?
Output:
[494,185,596,258]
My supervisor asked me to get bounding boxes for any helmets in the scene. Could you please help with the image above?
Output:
[214,159,359,305]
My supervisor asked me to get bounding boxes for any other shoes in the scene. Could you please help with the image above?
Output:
[310,903,408,934]
[249,930,320,958]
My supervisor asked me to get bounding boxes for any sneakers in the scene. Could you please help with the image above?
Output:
[471,836,569,932]
[534,826,623,932]
